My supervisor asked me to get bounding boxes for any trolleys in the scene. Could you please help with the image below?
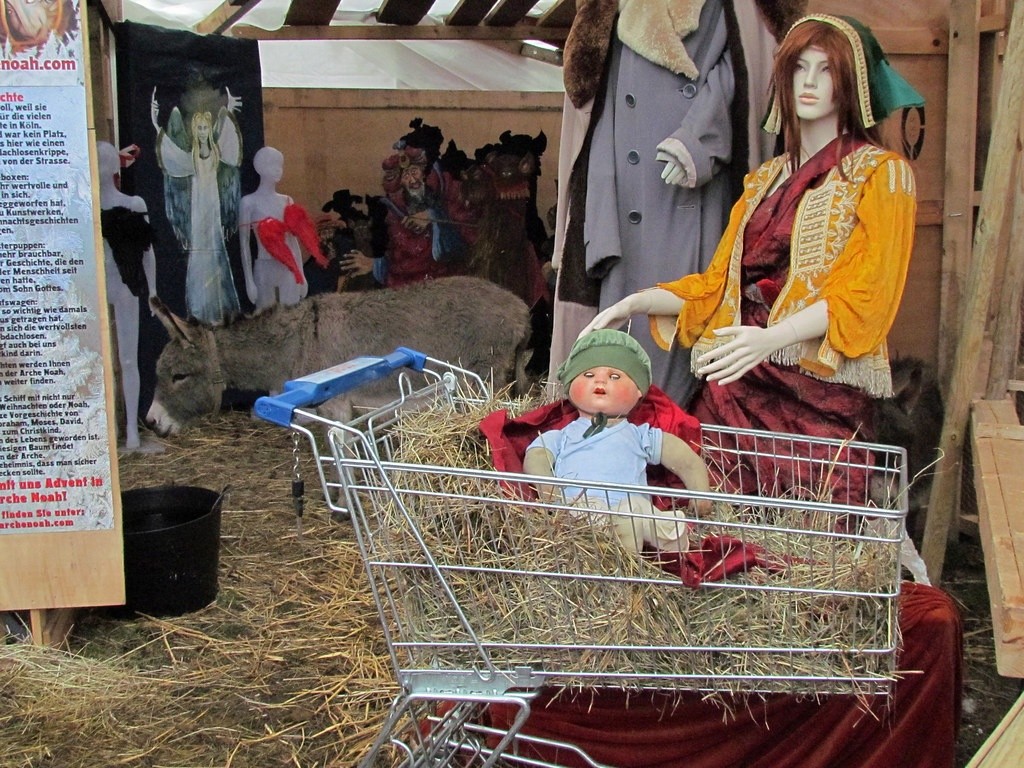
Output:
[255,346,907,768]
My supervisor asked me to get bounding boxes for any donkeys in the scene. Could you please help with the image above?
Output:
[140,279,535,528]
[868,355,978,519]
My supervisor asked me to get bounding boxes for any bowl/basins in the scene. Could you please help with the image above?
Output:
[105,483,234,619]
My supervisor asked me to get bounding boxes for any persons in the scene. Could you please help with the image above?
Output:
[95,141,157,450]
[524,328,710,554]
[238,147,308,311]
[553,0,783,409]
[580,14,923,524]
